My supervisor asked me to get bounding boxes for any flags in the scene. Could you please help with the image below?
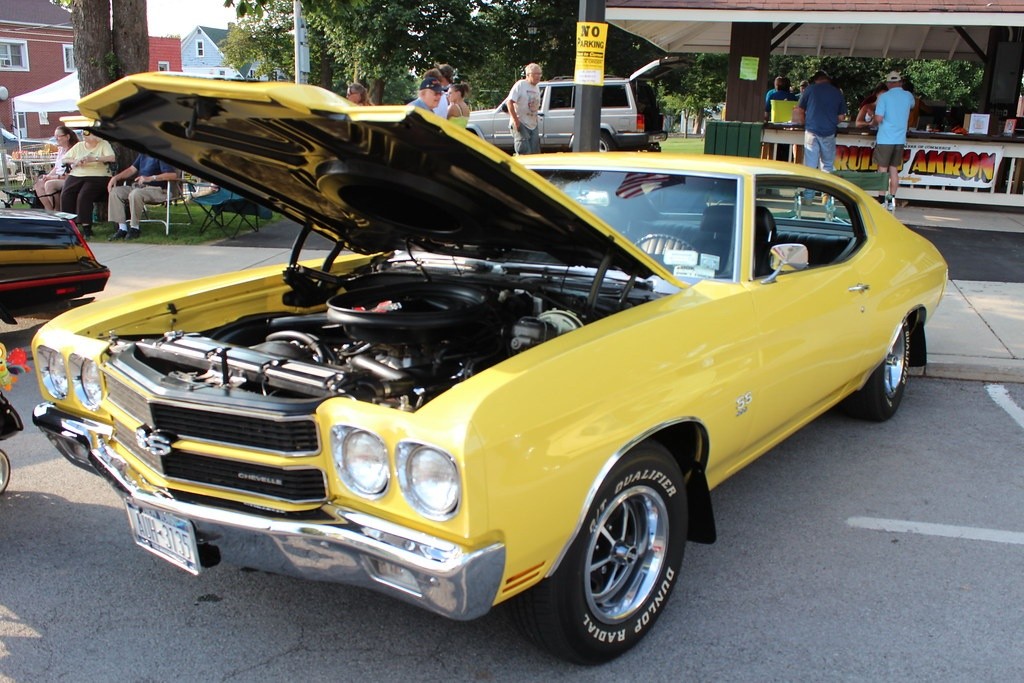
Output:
[615,173,686,199]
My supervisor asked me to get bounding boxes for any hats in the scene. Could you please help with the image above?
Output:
[419,78,445,92]
[887,71,901,81]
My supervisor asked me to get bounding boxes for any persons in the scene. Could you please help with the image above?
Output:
[797,71,847,206]
[406,64,471,130]
[107,152,178,241]
[855,85,889,129]
[764,75,809,124]
[59,129,116,235]
[34,126,79,212]
[506,63,542,155]
[902,79,920,129]
[346,83,376,107]
[869,71,916,212]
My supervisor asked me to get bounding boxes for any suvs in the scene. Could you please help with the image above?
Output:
[462,57,698,153]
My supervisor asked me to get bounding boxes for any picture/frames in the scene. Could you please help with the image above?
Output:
[969,114,991,134]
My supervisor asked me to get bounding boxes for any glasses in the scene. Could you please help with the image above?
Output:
[426,91,442,97]
[53,134,66,140]
[528,72,542,77]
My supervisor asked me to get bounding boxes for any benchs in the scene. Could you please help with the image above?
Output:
[645,222,849,271]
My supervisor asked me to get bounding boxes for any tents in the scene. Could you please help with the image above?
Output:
[12,71,82,180]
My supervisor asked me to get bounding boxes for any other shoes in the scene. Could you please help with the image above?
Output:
[821,194,829,205]
[887,201,895,211]
[801,199,813,205]
[880,203,888,210]
[125,228,141,241]
[108,229,128,241]
[83,230,94,240]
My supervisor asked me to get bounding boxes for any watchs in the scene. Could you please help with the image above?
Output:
[96,157,99,162]
[152,175,157,181]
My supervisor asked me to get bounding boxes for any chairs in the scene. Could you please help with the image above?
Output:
[0,169,47,209]
[699,205,780,268]
[116,166,193,236]
[193,178,271,240]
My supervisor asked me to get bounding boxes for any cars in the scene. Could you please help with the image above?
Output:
[30,71,951,664]
[50,129,82,147]
[0,127,50,156]
[0,207,111,308]
